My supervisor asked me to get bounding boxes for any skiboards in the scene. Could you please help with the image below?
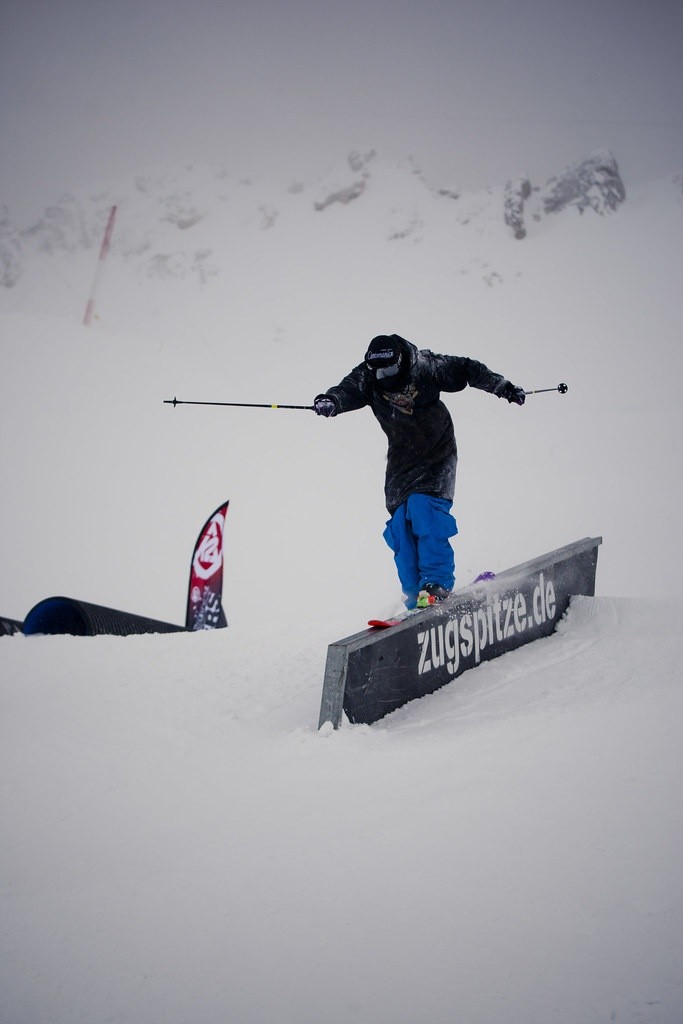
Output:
[368,570,495,627]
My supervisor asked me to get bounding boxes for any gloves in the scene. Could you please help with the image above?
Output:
[496,381,526,405]
[314,397,337,418]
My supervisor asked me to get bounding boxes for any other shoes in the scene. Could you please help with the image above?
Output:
[416,583,449,606]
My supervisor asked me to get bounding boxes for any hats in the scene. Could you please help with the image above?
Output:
[365,335,406,382]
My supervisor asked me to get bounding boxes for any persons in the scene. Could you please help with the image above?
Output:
[313,334,527,612]
